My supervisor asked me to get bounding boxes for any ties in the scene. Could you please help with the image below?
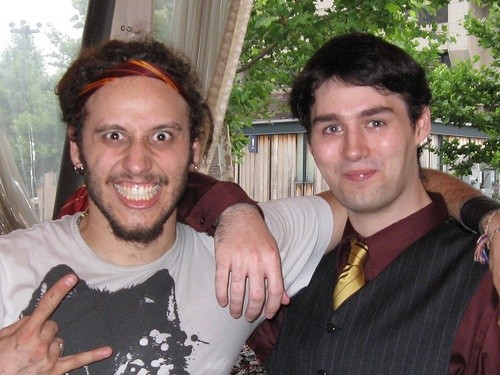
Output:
[332,235,369,311]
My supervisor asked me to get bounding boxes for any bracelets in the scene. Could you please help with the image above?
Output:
[475,209,500,261]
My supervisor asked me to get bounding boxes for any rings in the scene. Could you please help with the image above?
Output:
[50,339,64,356]
